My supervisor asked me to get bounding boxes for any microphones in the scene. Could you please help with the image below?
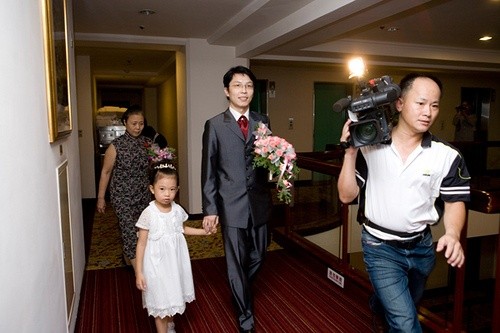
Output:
[333,97,349,113]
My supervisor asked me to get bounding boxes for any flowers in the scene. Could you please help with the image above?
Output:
[143,139,177,165]
[254,122,300,204]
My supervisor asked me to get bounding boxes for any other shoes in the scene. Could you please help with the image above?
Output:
[168,321,176,333]
[122,255,133,271]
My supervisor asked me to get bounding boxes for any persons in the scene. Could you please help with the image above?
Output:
[336,73,472,333]
[200,67,272,333]
[97,108,167,276]
[452,101,476,173]
[134,161,218,333]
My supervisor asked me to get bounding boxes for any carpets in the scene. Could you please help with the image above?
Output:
[86,195,285,271]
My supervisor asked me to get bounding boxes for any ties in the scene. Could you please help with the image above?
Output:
[239,116,250,140]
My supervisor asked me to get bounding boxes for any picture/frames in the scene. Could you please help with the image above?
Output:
[42,0,73,143]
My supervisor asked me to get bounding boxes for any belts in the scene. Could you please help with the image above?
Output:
[361,226,431,250]
